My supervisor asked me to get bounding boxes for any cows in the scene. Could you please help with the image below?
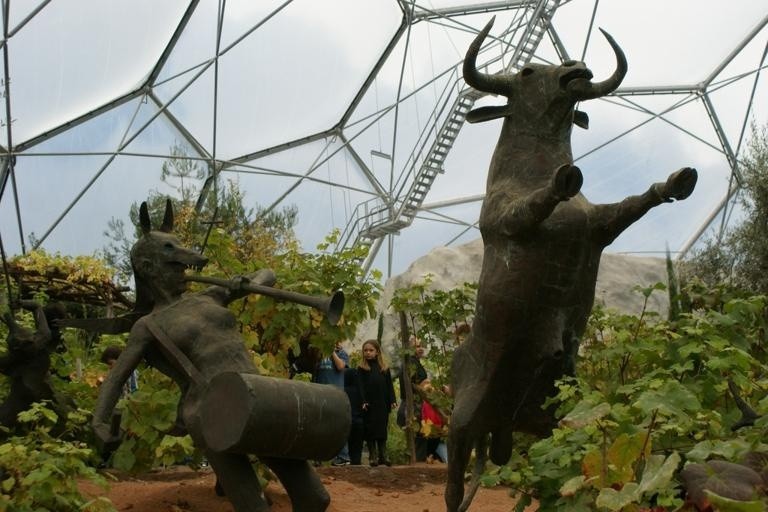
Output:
[444,14,698,512]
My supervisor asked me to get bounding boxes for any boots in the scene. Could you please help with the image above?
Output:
[377,440,391,465]
[367,441,378,466]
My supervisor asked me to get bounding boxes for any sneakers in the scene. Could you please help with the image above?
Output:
[331,457,350,465]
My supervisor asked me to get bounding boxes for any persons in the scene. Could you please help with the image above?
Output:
[356,338,395,465]
[92,345,140,401]
[399,336,441,461]
[317,342,351,466]
